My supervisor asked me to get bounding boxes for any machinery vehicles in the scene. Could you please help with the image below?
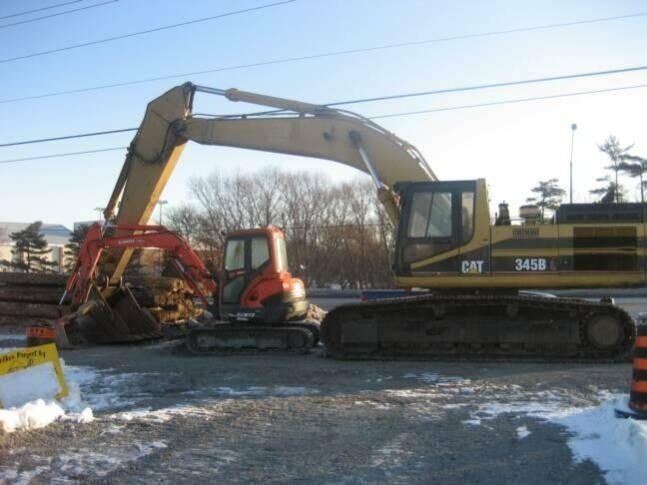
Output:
[26,222,319,353]
[55,81,647,364]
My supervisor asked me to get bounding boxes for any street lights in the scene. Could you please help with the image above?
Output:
[569,123,578,204]
[154,199,168,225]
[94,207,106,222]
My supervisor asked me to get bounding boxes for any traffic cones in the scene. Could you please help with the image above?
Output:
[613,311,647,422]
